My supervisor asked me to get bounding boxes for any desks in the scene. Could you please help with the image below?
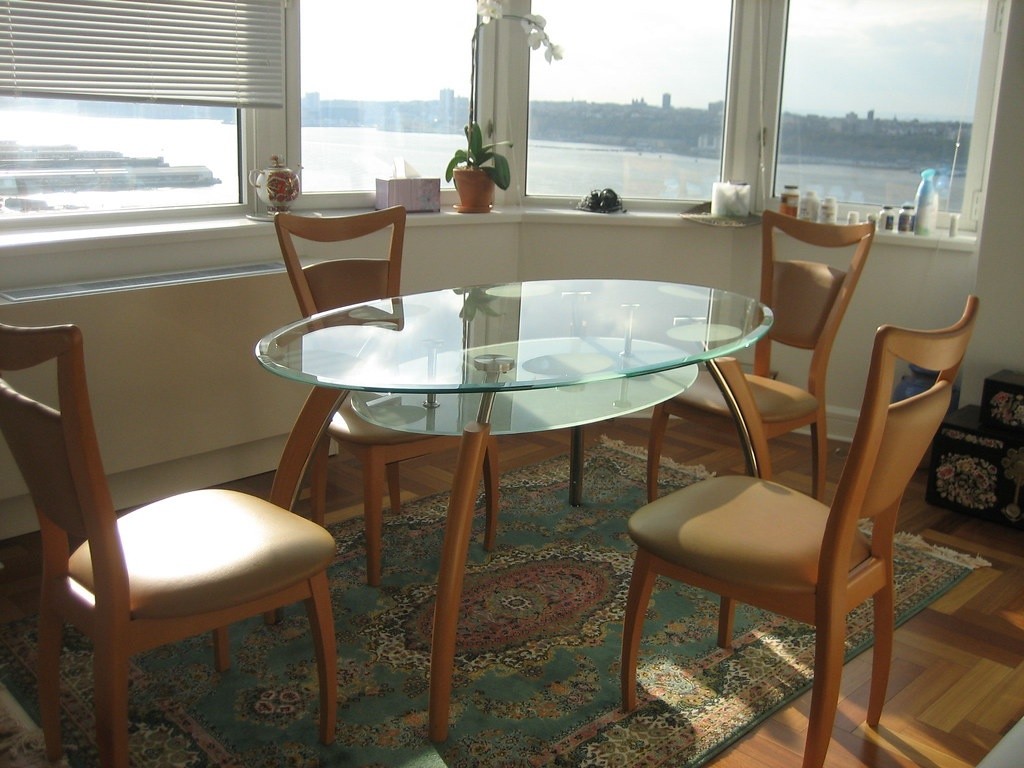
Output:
[253,278,777,745]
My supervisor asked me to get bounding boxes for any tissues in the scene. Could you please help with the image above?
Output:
[374,153,441,212]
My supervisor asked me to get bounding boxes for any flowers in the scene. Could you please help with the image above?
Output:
[446,0,563,192]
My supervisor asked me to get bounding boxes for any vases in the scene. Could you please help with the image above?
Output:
[449,167,495,212]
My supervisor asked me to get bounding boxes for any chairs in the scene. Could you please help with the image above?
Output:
[275,205,502,589]
[0,324,339,768]
[644,208,878,510]
[616,292,981,768]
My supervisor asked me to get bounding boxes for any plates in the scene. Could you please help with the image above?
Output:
[246,208,323,222]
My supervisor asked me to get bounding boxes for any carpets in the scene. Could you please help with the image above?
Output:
[1,435,991,767]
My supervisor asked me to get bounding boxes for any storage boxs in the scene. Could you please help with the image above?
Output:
[923,360,1024,531]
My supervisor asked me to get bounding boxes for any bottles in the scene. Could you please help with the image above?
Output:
[779,184,799,218]
[878,206,895,231]
[817,195,837,223]
[898,205,918,235]
[847,212,860,226]
[799,190,820,224]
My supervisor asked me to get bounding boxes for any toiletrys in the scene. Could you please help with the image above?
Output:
[777,168,940,236]
